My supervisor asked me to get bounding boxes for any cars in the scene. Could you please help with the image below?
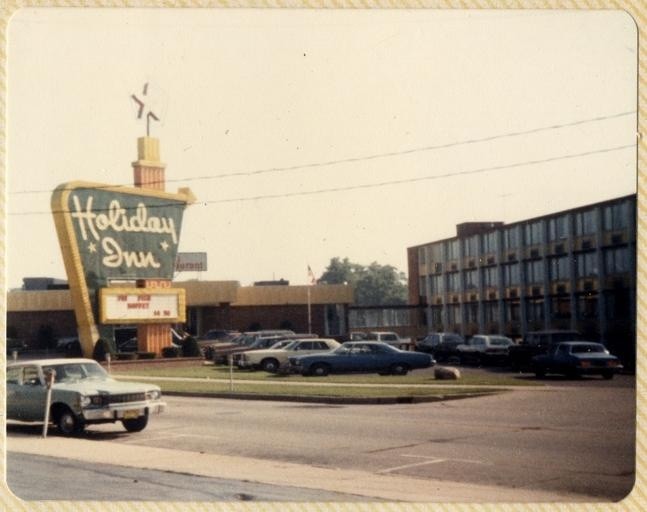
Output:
[456,335,516,366]
[509,330,624,378]
[9,329,181,359]
[179,329,436,376]
[417,332,464,362]
[7,359,167,435]
